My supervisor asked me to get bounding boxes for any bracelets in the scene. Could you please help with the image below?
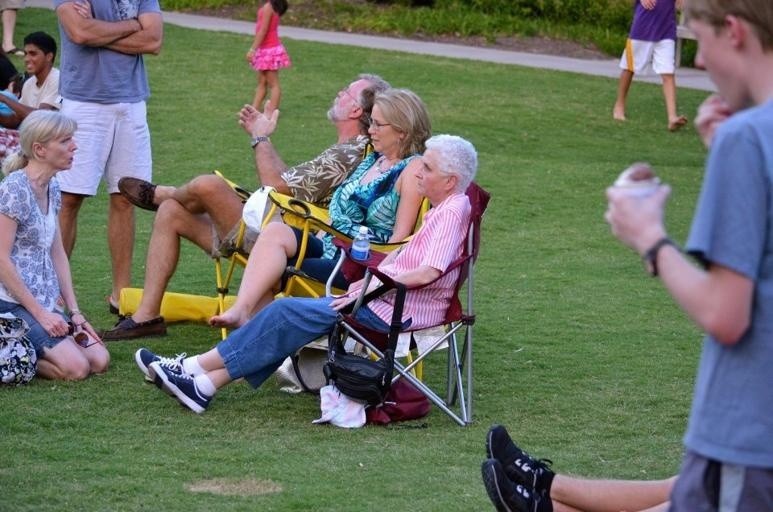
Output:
[249,47,256,53]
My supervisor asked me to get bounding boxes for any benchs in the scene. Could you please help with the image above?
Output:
[672,7,700,67]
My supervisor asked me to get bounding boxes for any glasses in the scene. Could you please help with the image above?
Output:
[367,117,391,130]
[65,319,108,348]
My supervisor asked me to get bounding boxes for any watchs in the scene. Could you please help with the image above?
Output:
[250,136,269,151]
[640,237,674,279]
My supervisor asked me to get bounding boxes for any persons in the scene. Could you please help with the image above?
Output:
[0,0,110,381]
[246,1,289,121]
[208,87,432,330]
[52,0,163,315]
[94,71,391,342]
[603,0,772,511]
[613,0,689,131]
[481,423,682,512]
[135,135,479,415]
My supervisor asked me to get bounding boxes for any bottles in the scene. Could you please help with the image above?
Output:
[351,226,370,261]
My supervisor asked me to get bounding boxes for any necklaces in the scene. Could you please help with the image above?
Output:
[375,156,395,174]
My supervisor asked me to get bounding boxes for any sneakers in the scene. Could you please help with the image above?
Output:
[148,360,216,416]
[134,347,195,380]
[484,423,553,497]
[481,458,542,512]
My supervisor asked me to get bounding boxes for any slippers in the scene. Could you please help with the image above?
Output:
[5,47,26,57]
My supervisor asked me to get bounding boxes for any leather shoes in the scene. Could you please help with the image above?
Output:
[93,314,167,342]
[117,176,159,212]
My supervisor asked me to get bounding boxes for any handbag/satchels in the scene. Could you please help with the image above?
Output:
[0,312,37,387]
[322,341,399,407]
